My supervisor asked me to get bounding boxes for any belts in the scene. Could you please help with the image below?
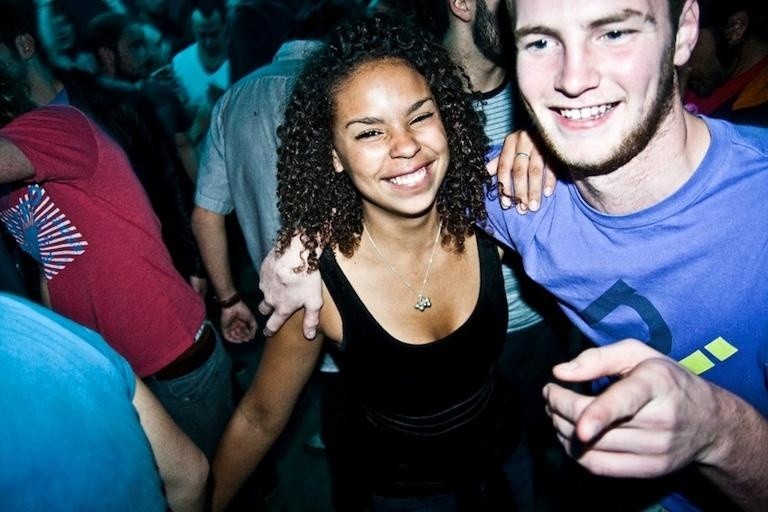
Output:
[141,322,217,386]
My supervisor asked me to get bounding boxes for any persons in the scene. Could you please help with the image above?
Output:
[0,1,768,511]
